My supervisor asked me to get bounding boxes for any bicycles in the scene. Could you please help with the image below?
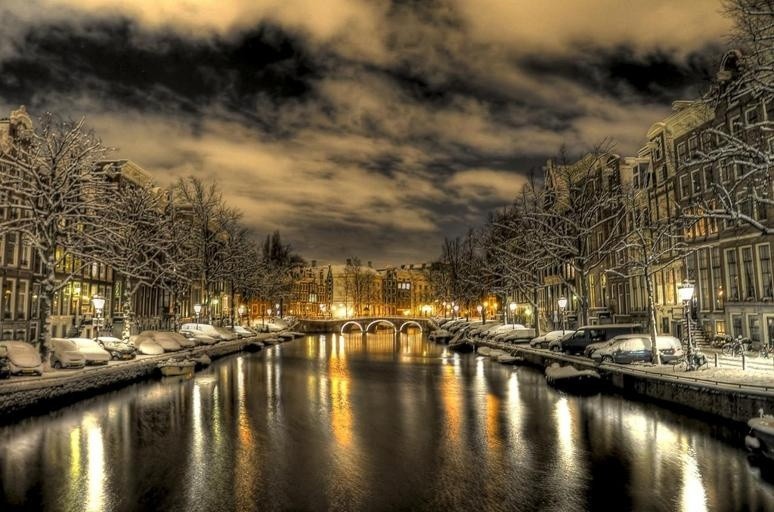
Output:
[759,338,774,359]
[672,350,709,373]
[721,334,746,357]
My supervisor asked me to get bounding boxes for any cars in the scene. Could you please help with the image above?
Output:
[0,340,44,376]
[440,319,685,366]
[44,316,294,370]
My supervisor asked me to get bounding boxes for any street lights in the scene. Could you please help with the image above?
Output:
[558,296,567,336]
[194,304,201,329]
[238,308,243,326]
[91,295,106,343]
[677,284,696,372]
[266,309,271,324]
[510,302,517,330]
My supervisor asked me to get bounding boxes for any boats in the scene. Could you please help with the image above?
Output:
[545,363,609,397]
